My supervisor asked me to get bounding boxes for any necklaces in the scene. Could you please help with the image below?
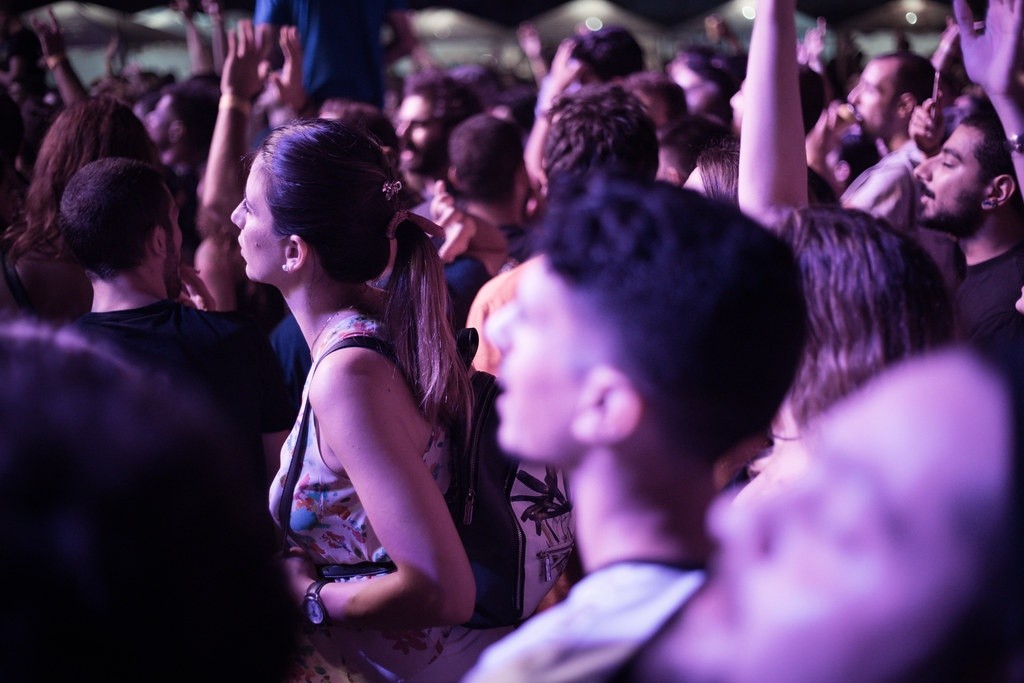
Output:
[310,302,357,362]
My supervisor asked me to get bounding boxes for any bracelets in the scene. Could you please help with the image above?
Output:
[218,95,251,116]
[46,53,67,69]
[535,106,551,118]
[1006,132,1024,154]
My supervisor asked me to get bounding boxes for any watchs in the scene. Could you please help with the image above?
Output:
[302,581,339,631]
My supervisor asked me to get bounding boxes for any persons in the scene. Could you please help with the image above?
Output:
[1,0,1024,683]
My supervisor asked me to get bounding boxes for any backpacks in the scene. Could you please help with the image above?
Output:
[277,326,527,632]
